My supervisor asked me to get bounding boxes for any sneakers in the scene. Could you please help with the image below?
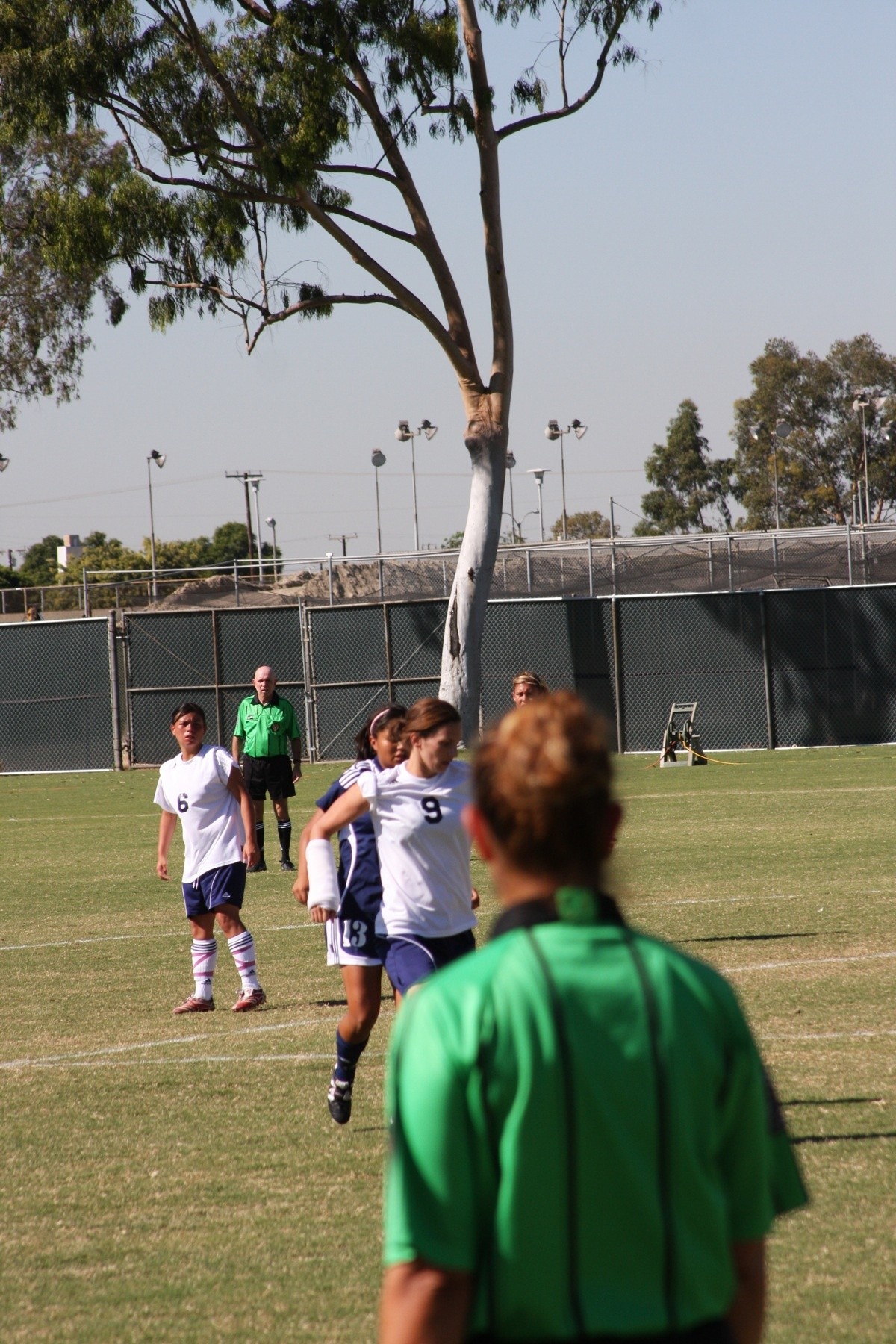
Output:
[172,996,215,1015]
[327,1063,356,1125]
[246,861,267,873]
[231,986,267,1012]
[281,860,296,871]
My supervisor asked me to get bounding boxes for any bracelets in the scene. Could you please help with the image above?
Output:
[293,759,301,764]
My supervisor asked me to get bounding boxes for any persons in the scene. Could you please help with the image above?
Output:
[152,703,268,1012]
[510,670,548,710]
[290,697,480,1127]
[26,602,39,622]
[231,666,302,873]
[374,686,812,1344]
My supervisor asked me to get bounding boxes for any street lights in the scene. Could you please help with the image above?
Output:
[771,418,792,530]
[544,418,588,540]
[506,452,517,544]
[395,419,439,552]
[526,468,548,542]
[371,448,386,554]
[243,476,265,585]
[502,510,540,543]
[147,449,167,595]
[852,388,890,525]
[265,517,278,583]
